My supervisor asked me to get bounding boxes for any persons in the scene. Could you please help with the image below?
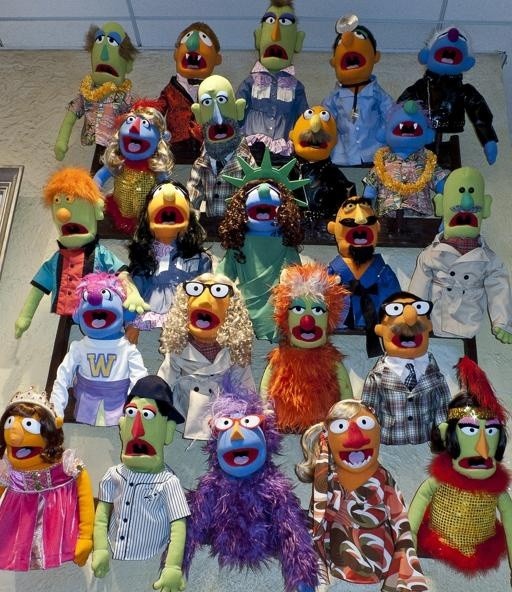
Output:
[0,375,510,591]
[53,1,499,248]
[15,166,510,445]
